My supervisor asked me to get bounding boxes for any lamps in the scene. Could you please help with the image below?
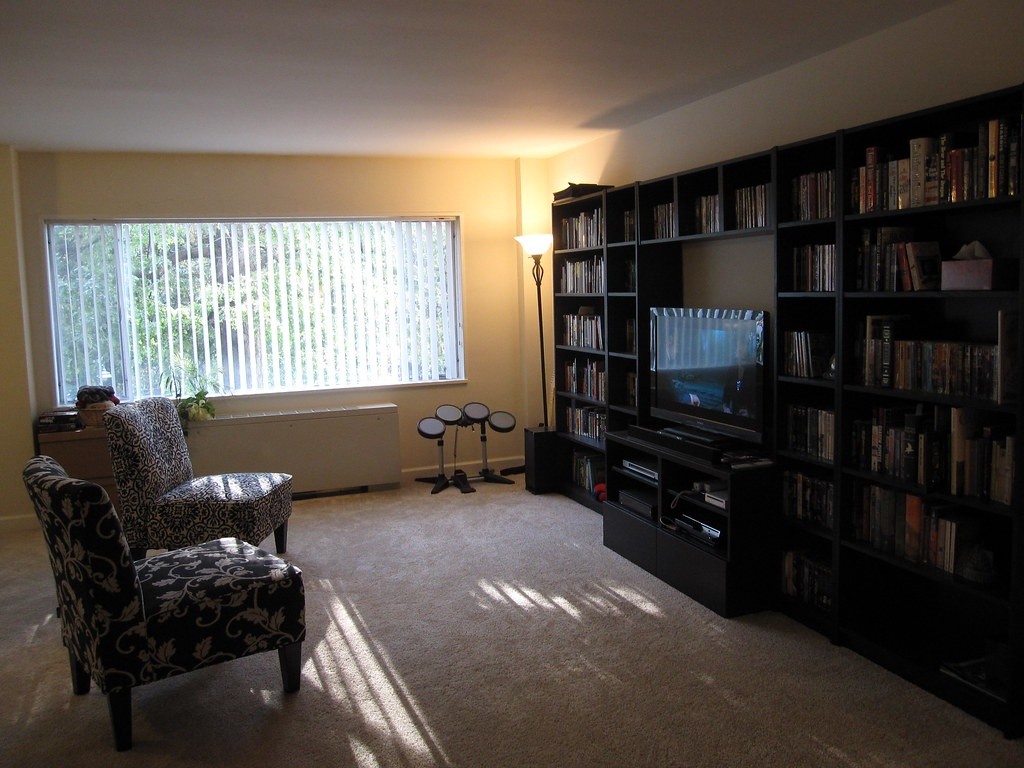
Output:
[514,233,557,497]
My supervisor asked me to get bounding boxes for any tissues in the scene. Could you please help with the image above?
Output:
[940,240,993,291]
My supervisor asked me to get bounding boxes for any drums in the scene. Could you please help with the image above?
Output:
[434,404,462,425]
[463,402,490,422]
[488,411,516,433]
[417,417,446,439]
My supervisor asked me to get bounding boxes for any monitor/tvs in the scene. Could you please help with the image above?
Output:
[649,308,770,446]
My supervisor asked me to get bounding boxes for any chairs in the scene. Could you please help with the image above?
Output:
[101,396,294,560]
[20,454,307,752]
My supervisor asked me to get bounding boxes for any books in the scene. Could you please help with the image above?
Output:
[558,208,636,495]
[652,118,1024,613]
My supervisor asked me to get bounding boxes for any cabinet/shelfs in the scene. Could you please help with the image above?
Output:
[550,83,1022,740]
[603,430,777,619]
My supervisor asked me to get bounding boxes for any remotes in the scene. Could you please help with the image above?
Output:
[720,454,773,469]
